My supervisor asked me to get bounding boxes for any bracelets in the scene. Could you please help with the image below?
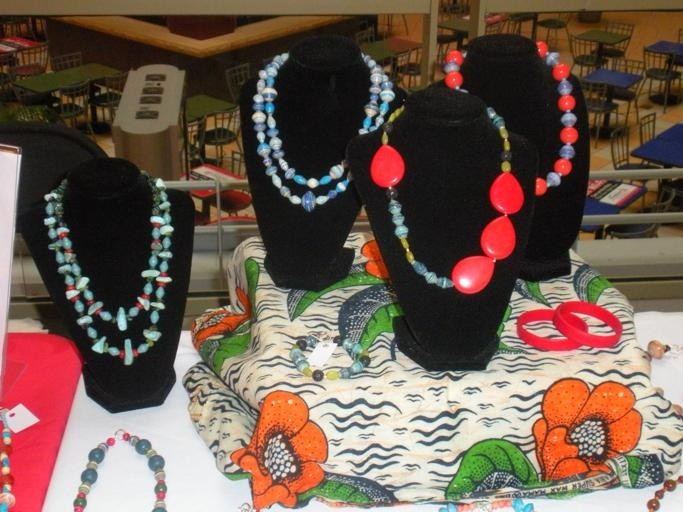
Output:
[516,308,587,353]
[289,330,370,383]
[553,301,623,349]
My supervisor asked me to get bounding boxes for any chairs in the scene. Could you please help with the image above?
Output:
[1,14,274,241]
[357,14,568,91]
[569,14,683,245]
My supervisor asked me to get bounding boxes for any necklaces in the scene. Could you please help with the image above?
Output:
[261,53,384,189]
[45,168,174,366]
[50,172,162,332]
[73,426,166,512]
[368,86,524,297]
[645,474,681,512]
[438,496,533,512]
[442,40,577,198]
[0,405,15,512]
[248,47,395,214]
